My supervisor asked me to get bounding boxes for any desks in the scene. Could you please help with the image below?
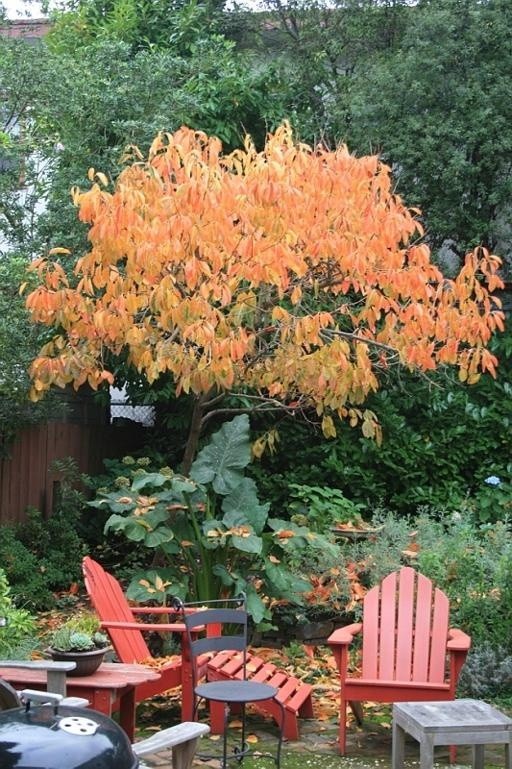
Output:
[2,653,161,743]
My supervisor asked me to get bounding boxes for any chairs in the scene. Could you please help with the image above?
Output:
[326,561,472,757]
[171,589,291,768]
[0,658,211,768]
[77,555,226,738]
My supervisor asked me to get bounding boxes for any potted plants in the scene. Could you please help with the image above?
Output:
[41,611,119,678]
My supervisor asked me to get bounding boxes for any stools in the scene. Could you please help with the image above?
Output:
[392,698,512,769]
